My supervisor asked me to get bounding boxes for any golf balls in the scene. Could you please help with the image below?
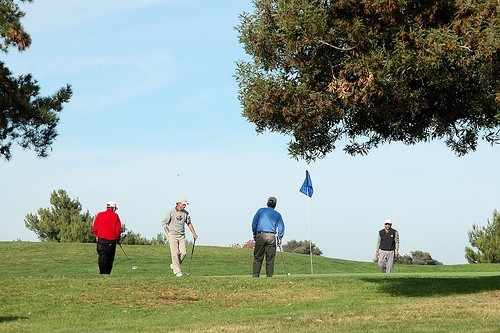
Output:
[288,273,291,275]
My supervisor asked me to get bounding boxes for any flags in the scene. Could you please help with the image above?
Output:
[299,170,313,198]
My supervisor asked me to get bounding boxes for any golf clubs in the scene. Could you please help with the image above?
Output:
[396,259,401,273]
[278,245,291,276]
[186,238,196,275]
[117,241,137,270]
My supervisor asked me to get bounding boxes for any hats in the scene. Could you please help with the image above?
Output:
[176,198,189,204]
[106,201,118,210]
[268,197,276,202]
[384,219,392,223]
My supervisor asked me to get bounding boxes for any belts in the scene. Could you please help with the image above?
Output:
[256,232,276,234]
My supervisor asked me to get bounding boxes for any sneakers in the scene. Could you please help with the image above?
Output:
[176,272,183,276]
[170,264,176,274]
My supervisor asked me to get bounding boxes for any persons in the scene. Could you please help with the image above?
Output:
[252,197,285,277]
[376,219,399,273]
[93,201,122,275]
[162,199,198,276]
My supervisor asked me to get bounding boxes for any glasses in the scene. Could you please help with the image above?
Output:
[385,223,392,225]
